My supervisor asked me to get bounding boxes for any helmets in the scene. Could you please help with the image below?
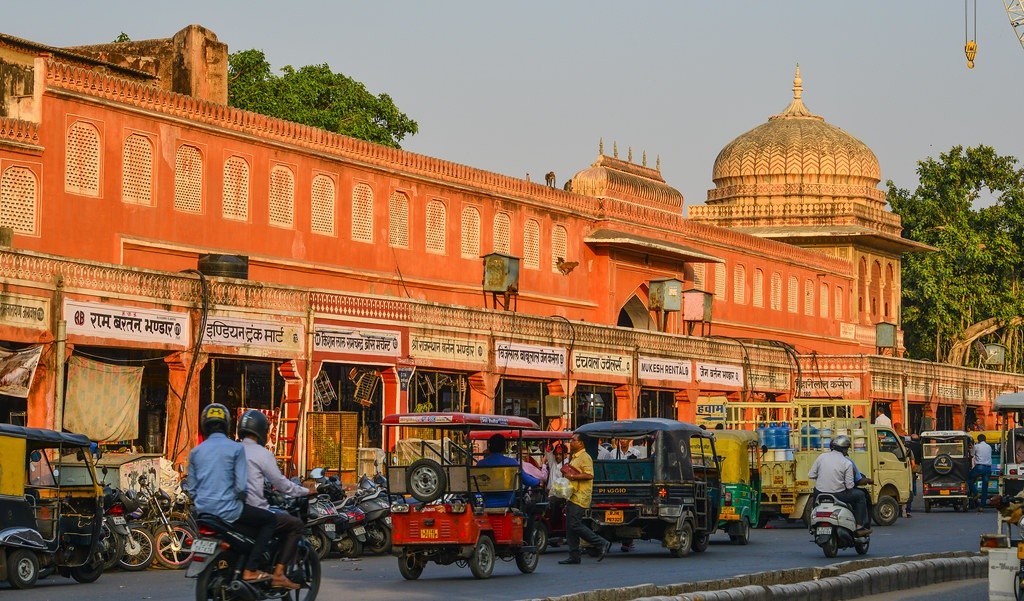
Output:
[200,402,231,434]
[832,434,851,448]
[238,409,271,445]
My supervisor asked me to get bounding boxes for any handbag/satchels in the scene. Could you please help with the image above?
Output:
[547,476,574,503]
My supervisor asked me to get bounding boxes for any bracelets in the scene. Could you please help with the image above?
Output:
[570,474,574,481]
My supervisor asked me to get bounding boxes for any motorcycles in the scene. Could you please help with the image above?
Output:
[690,427,763,545]
[572,416,723,558]
[968,431,1007,495]
[380,413,543,579]
[920,431,978,512]
[463,430,574,555]
[184,495,322,601]
[0,422,108,588]
[102,466,391,571]
[1006,428,1024,493]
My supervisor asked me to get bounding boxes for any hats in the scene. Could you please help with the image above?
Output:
[487,434,507,450]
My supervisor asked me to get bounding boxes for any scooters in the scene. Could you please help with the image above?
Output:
[809,473,878,557]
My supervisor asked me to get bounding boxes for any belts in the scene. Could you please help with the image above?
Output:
[977,464,991,468]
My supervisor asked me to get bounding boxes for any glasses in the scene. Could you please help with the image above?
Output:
[567,438,582,444]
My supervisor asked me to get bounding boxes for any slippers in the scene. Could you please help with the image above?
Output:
[247,571,273,583]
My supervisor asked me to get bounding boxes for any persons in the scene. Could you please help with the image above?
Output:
[474,432,541,546]
[874,406,892,429]
[234,410,317,591]
[808,434,876,537]
[508,439,647,498]
[874,404,993,519]
[555,430,612,564]
[184,400,281,584]
[969,433,992,514]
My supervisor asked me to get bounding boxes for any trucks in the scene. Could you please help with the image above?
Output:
[694,396,913,525]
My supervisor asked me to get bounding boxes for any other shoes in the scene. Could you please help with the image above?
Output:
[271,578,301,590]
[558,557,581,564]
[978,507,984,512]
[597,541,612,563]
[906,512,912,518]
[856,527,872,537]
[973,494,981,502]
